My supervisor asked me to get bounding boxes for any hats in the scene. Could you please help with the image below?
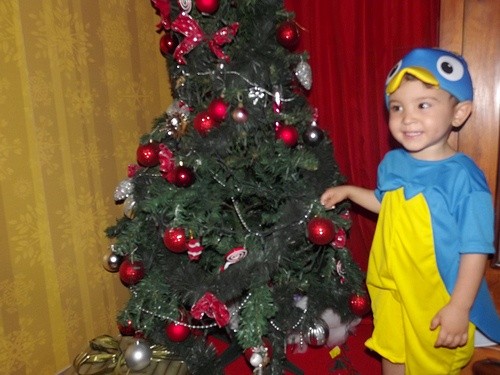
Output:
[384,48,474,110]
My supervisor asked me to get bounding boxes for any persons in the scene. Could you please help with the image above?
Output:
[321,45,499,374]
